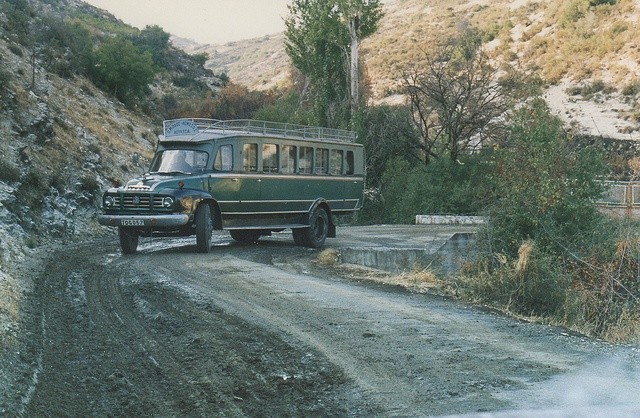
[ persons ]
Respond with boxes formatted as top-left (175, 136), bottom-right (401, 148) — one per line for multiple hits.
top-left (169, 150), bottom-right (193, 174)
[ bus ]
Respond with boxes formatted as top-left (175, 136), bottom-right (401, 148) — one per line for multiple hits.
top-left (99, 117), bottom-right (366, 255)
